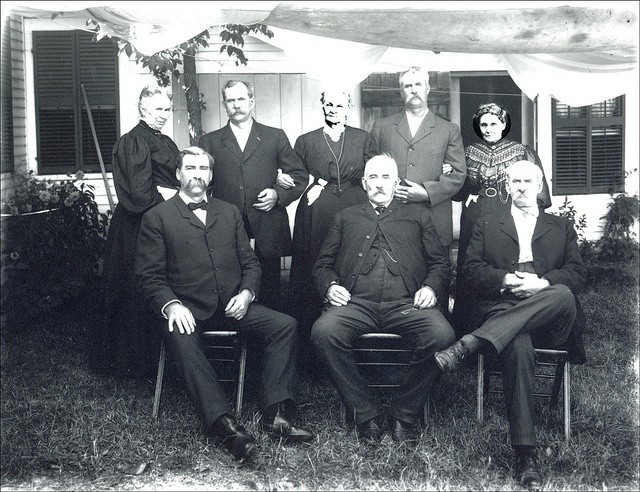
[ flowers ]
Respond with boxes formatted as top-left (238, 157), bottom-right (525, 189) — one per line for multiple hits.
top-left (2, 170), bottom-right (107, 269)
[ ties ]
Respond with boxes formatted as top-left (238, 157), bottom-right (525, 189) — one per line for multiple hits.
top-left (374, 205), bottom-right (386, 215)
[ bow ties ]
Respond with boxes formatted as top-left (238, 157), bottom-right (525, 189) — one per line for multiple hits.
top-left (187, 200), bottom-right (207, 210)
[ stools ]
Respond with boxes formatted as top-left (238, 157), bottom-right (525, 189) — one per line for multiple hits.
top-left (477, 348), bottom-right (570, 441)
top-left (340, 333), bottom-right (429, 428)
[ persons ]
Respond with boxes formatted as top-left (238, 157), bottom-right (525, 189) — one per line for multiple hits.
top-left (89, 85), bottom-right (184, 380)
top-left (200, 78), bottom-right (309, 316)
top-left (440, 101), bottom-right (550, 341)
top-left (367, 62), bottom-right (467, 321)
top-left (128, 145), bottom-right (312, 465)
top-left (433, 160), bottom-right (588, 488)
top-left (308, 152), bottom-right (455, 452)
top-left (276, 89), bottom-right (383, 381)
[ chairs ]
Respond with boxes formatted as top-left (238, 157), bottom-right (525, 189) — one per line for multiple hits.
top-left (151, 239), bottom-right (256, 420)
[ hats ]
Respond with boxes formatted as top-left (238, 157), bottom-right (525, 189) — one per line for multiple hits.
top-left (473, 104), bottom-right (512, 140)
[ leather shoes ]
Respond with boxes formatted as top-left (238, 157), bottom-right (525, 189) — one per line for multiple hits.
top-left (213, 415), bottom-right (257, 460)
top-left (388, 415), bottom-right (416, 447)
top-left (258, 406), bottom-right (312, 442)
top-left (515, 454), bottom-right (542, 487)
top-left (359, 418), bottom-right (383, 445)
top-left (435, 342), bottom-right (468, 376)
